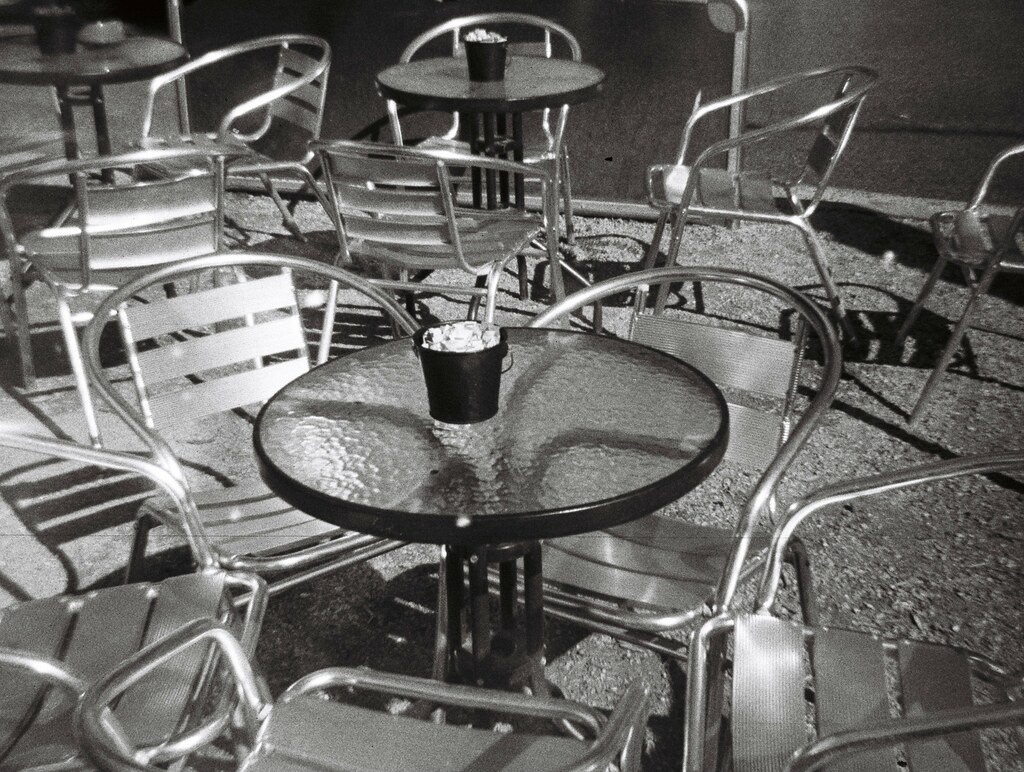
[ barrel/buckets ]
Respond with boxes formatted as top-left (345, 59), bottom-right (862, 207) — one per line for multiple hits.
top-left (33, 11), bottom-right (78, 54)
top-left (462, 36), bottom-right (511, 82)
top-left (413, 320), bottom-right (514, 425)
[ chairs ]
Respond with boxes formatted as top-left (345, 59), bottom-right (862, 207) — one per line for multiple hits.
top-left (639, 63), bottom-right (886, 352)
top-left (1, 134), bottom-right (99, 391)
top-left (1, 434), bottom-right (269, 772)
top-left (78, 615), bottom-right (652, 772)
top-left (80, 252), bottom-right (430, 712)
top-left (2, 142), bottom-right (255, 400)
top-left (682, 452), bottom-right (1023, 772)
top-left (888, 140), bottom-right (1024, 428)
top-left (136, 34), bottom-right (361, 244)
top-left (437, 263), bottom-right (845, 709)
top-left (305, 138), bottom-right (575, 339)
top-left (384, 12), bottom-right (584, 243)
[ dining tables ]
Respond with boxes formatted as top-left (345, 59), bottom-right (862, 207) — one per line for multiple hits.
top-left (374, 56), bottom-right (606, 299)
top-left (1, 33), bottom-right (189, 190)
top-left (253, 327), bottom-right (730, 743)
